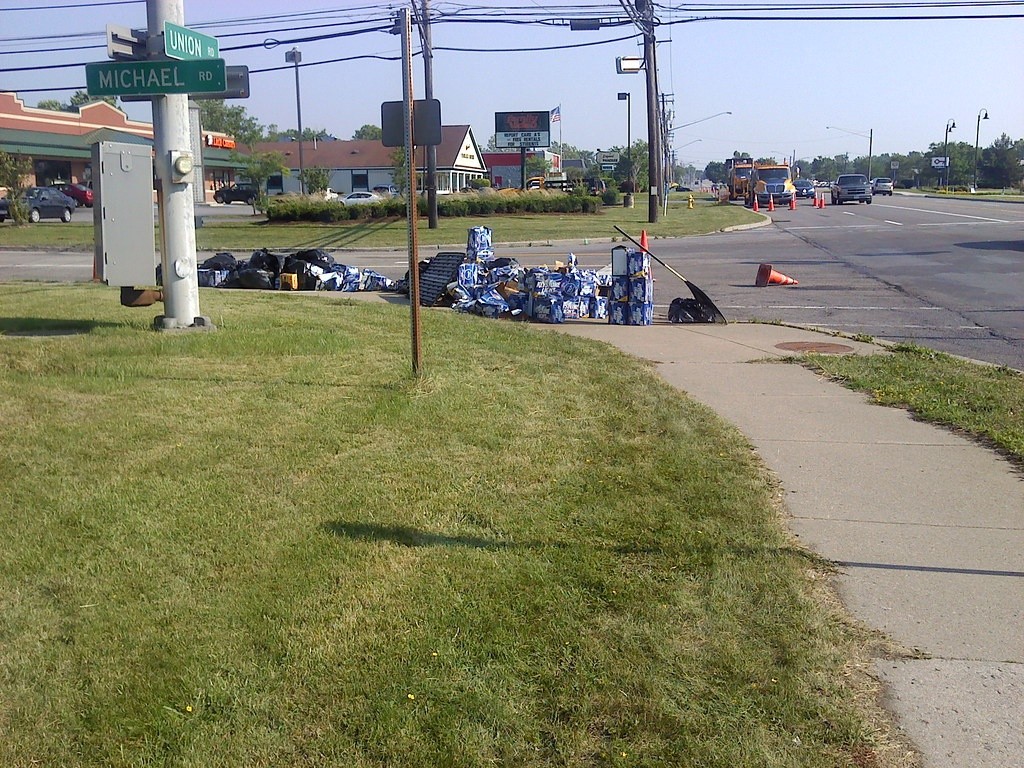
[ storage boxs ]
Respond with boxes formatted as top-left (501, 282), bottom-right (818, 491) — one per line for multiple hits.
top-left (198, 263), bottom-right (394, 292)
top-left (445, 225), bottom-right (654, 327)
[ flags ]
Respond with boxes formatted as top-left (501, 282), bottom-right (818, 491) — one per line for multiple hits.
top-left (550, 106), bottom-right (560, 122)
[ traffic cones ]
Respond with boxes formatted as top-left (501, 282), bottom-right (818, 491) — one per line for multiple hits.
top-left (639, 231), bottom-right (656, 282)
top-left (753, 193), bottom-right (826, 212)
top-left (755, 265), bottom-right (799, 285)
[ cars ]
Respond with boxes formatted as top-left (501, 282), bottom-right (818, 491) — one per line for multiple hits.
top-left (337, 192), bottom-right (382, 204)
top-left (313, 189), bottom-right (340, 200)
top-left (48, 184), bottom-right (94, 208)
top-left (81, 181), bottom-right (93, 189)
top-left (792, 180), bottom-right (815, 199)
top-left (0, 188), bottom-right (76, 223)
top-left (813, 182), bottom-right (835, 187)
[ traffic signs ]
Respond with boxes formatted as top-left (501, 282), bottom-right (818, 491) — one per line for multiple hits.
top-left (85, 58), bottom-right (227, 96)
top-left (163, 21), bottom-right (219, 60)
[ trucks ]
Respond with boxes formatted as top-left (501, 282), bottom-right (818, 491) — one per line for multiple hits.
top-left (745, 164), bottom-right (797, 205)
top-left (725, 158), bottom-right (755, 200)
top-left (519, 177), bottom-right (606, 195)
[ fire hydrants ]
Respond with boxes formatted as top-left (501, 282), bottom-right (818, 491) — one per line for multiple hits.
top-left (687, 194), bottom-right (695, 209)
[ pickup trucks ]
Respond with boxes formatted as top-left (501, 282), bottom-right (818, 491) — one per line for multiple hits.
top-left (830, 173), bottom-right (872, 205)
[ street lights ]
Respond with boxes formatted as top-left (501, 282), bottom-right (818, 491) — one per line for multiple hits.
top-left (827, 127), bottom-right (871, 184)
top-left (285, 50), bottom-right (306, 194)
top-left (974, 108), bottom-right (990, 186)
top-left (618, 92), bottom-right (632, 195)
top-left (943, 118), bottom-right (957, 188)
top-left (664, 111), bottom-right (732, 186)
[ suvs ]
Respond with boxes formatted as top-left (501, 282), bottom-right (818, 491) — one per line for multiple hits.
top-left (213, 183), bottom-right (261, 205)
top-left (373, 184), bottom-right (399, 198)
top-left (871, 178), bottom-right (893, 196)
